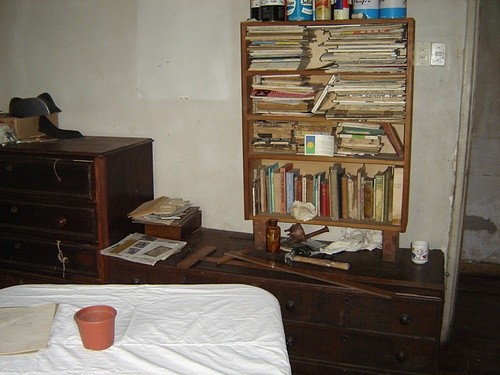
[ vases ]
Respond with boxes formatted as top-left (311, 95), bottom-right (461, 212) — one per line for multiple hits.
top-left (74, 305), bottom-right (118, 351)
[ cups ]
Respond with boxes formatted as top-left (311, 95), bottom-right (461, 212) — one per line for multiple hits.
top-left (75, 305), bottom-right (117, 351)
top-left (411, 241), bottom-right (429, 265)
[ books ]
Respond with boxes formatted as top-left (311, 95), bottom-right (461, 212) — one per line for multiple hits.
top-left (246, 25), bottom-right (408, 225)
top-left (127, 196), bottom-right (192, 226)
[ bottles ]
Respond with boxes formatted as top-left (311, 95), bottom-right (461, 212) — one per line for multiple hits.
top-left (266, 219), bottom-right (281, 254)
top-left (249, 0), bottom-right (408, 21)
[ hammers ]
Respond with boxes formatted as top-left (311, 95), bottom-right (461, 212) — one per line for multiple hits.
top-left (284, 252), bottom-right (351, 270)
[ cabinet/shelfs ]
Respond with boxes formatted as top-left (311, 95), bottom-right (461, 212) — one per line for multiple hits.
top-left (0, 133), bottom-right (155, 285)
top-left (108, 222), bottom-right (446, 374)
top-left (238, 17), bottom-right (417, 263)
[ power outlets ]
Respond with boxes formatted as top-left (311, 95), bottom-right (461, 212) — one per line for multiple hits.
top-left (430, 42), bottom-right (448, 67)
top-left (414, 41), bottom-right (431, 68)
